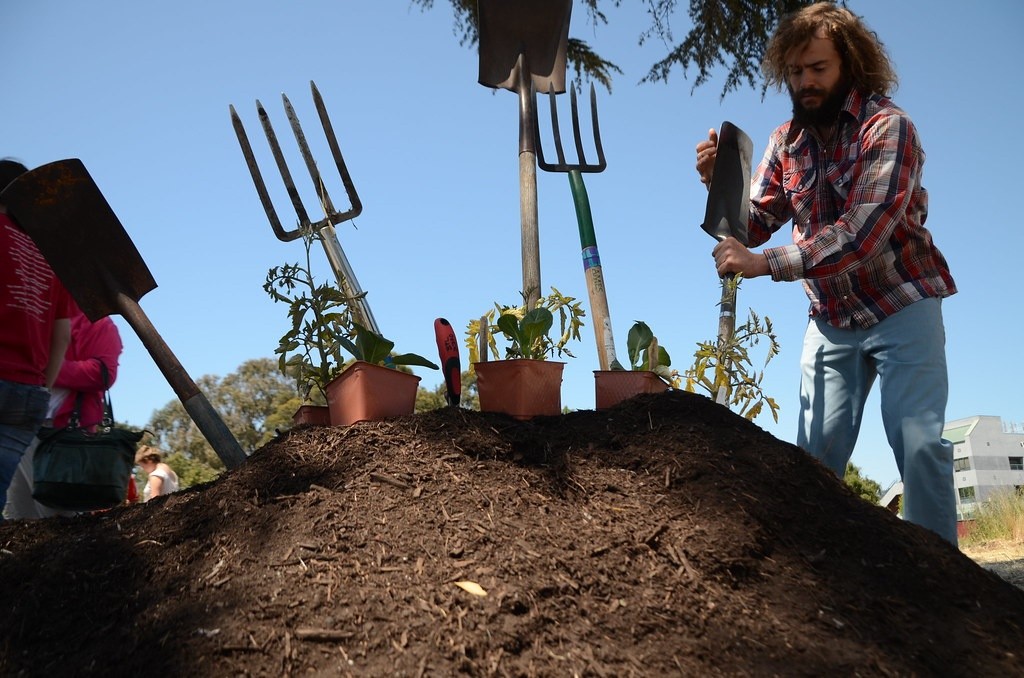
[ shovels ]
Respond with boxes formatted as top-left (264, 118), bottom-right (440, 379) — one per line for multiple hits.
top-left (470, 0), bottom-right (573, 360)
top-left (701, 121), bottom-right (755, 405)
top-left (0, 157), bottom-right (250, 472)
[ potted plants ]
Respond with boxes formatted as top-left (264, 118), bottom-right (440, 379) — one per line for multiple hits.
top-left (321, 321), bottom-right (439, 425)
top-left (592, 321), bottom-right (672, 410)
top-left (470, 307), bottom-right (568, 422)
top-left (262, 220), bottom-right (368, 425)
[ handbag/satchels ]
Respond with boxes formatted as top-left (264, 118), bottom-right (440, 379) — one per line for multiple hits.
top-left (31, 425), bottom-right (147, 511)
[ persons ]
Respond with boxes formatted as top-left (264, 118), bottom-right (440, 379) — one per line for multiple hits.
top-left (0, 157), bottom-right (180, 517)
top-left (695, 2), bottom-right (959, 547)
